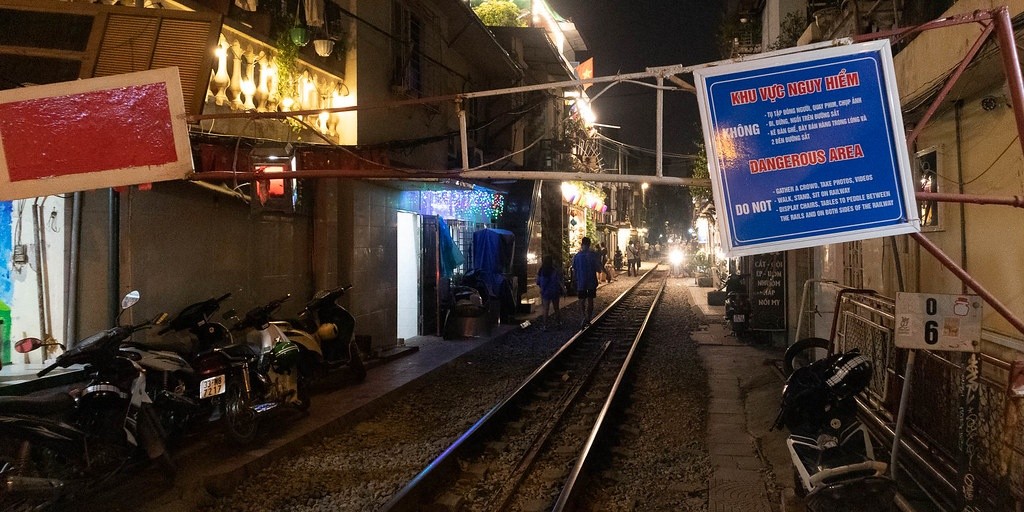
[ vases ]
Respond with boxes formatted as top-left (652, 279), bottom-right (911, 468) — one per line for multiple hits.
top-left (312, 39), bottom-right (336, 57)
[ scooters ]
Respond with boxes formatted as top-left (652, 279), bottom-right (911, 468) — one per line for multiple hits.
top-left (718, 270), bottom-right (751, 340)
top-left (114, 282), bottom-right (235, 443)
top-left (193, 294), bottom-right (309, 447)
top-left (269, 283), bottom-right (367, 393)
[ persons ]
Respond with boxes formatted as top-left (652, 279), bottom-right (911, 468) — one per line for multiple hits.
top-left (647, 242), bottom-right (665, 259)
top-left (537, 255), bottom-right (566, 324)
top-left (626, 240), bottom-right (643, 277)
top-left (573, 236), bottom-right (611, 322)
top-left (666, 243), bottom-right (686, 277)
top-left (596, 242), bottom-right (608, 284)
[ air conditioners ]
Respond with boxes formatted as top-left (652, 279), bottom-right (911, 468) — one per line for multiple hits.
top-left (448, 136), bottom-right (458, 163)
top-left (468, 147), bottom-right (484, 168)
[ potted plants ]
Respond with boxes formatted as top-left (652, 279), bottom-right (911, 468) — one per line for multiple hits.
top-left (808, 0), bottom-right (841, 34)
top-left (697, 260), bottom-right (713, 288)
top-left (681, 254), bottom-right (707, 285)
top-left (275, 26), bottom-right (313, 99)
top-left (707, 259), bottom-right (728, 306)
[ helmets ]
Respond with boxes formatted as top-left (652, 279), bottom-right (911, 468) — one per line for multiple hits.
top-left (274, 342), bottom-right (302, 368)
top-left (318, 322), bottom-right (338, 342)
top-left (826, 348), bottom-right (872, 398)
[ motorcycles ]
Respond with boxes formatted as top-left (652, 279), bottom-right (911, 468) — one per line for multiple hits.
top-left (0, 290), bottom-right (168, 511)
